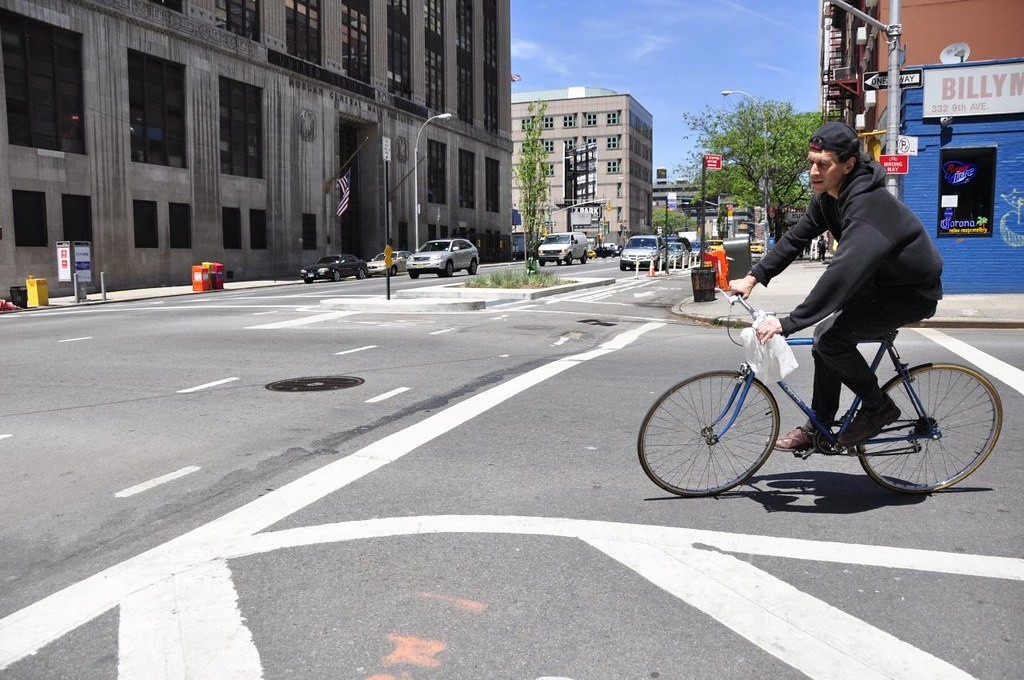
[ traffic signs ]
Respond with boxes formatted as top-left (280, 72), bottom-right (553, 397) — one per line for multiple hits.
top-left (862, 67), bottom-right (924, 91)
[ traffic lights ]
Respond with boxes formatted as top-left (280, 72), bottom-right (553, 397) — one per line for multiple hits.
top-left (607, 202), bottom-right (612, 211)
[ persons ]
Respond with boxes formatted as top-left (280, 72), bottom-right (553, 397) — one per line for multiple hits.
top-left (728, 120), bottom-right (944, 452)
top-left (818, 235), bottom-right (828, 262)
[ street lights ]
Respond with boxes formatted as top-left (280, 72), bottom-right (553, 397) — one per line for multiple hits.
top-left (673, 202), bottom-right (689, 235)
top-left (721, 90), bottom-right (768, 257)
top-left (415, 113), bottom-right (452, 252)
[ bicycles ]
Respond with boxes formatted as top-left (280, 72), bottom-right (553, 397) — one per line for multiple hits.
top-left (637, 288), bottom-right (1004, 497)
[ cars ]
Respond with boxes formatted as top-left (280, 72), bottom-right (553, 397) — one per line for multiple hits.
top-left (665, 234), bottom-right (723, 268)
top-left (750, 237), bottom-right (774, 253)
top-left (587, 246), bottom-right (620, 259)
top-left (367, 250), bottom-right (413, 278)
top-left (301, 254), bottom-right (367, 283)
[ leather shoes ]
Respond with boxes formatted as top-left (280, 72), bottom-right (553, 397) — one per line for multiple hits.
top-left (837, 392), bottom-right (901, 446)
top-left (767, 426), bottom-right (829, 452)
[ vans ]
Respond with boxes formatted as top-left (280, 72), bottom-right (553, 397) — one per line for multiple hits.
top-left (538, 232), bottom-right (588, 266)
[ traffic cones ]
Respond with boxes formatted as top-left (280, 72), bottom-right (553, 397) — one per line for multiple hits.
top-left (646, 257), bottom-right (658, 277)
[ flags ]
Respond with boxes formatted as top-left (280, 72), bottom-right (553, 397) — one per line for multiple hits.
top-left (335, 170), bottom-right (350, 216)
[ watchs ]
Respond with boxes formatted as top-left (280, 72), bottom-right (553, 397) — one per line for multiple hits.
top-left (747, 271), bottom-right (759, 282)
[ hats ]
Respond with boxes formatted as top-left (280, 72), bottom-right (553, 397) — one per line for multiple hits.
top-left (808, 121), bottom-right (871, 162)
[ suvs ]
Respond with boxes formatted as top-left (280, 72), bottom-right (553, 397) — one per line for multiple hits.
top-left (620, 234), bottom-right (667, 271)
top-left (406, 238), bottom-right (480, 279)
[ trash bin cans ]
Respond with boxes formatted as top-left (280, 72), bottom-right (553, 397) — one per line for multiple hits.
top-left (691, 267), bottom-right (716, 303)
top-left (10, 285), bottom-right (28, 309)
